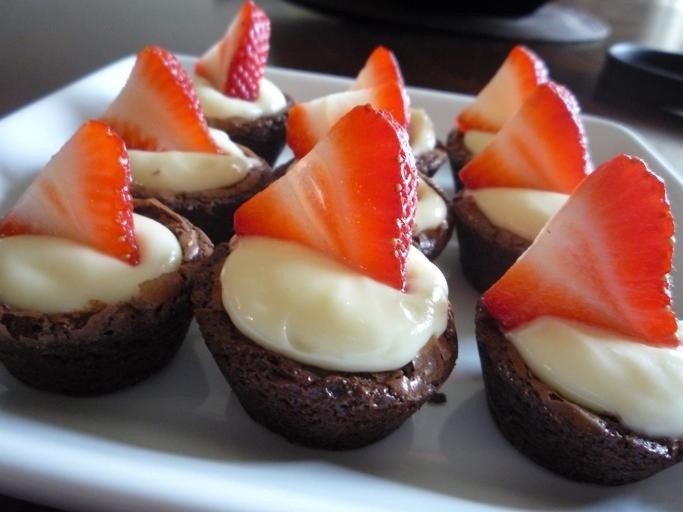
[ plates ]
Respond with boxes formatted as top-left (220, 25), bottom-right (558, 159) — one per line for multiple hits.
top-left (1, 52), bottom-right (682, 511)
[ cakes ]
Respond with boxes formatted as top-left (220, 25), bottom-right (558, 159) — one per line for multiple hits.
top-left (0, 120), bottom-right (215, 398)
top-left (98, 44), bottom-right (274, 244)
top-left (191, 103), bottom-right (458, 452)
top-left (190, 1), bottom-right (294, 171)
top-left (267, 82), bottom-right (457, 263)
top-left (475, 154), bottom-right (682, 486)
top-left (346, 43), bottom-right (449, 177)
top-left (447, 44), bottom-right (551, 168)
top-left (456, 79), bottom-right (595, 293)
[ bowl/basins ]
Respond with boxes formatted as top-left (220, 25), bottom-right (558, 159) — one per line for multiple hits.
top-left (604, 41), bottom-right (681, 117)
